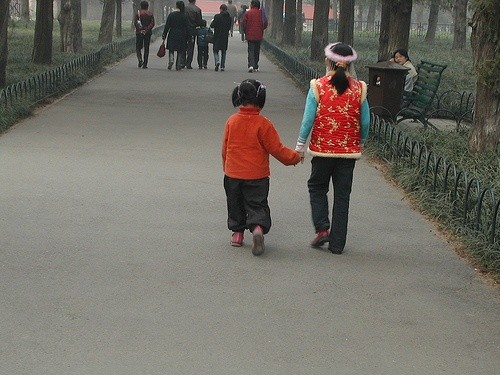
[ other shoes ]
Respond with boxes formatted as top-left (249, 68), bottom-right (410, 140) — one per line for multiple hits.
top-left (215, 63), bottom-right (220, 71)
top-left (139, 62), bottom-right (142, 67)
top-left (311, 231), bottom-right (330, 246)
top-left (143, 67), bottom-right (147, 68)
top-left (187, 67), bottom-right (192, 69)
top-left (199, 66), bottom-right (202, 69)
top-left (248, 67), bottom-right (253, 72)
top-left (254, 69), bottom-right (259, 72)
top-left (252, 226), bottom-right (265, 256)
top-left (230, 232), bottom-right (243, 246)
top-left (221, 68), bottom-right (224, 70)
top-left (204, 65), bottom-right (207, 69)
top-left (167, 62), bottom-right (173, 69)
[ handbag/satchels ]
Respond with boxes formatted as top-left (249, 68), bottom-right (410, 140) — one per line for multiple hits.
top-left (157, 41), bottom-right (165, 58)
top-left (205, 27), bottom-right (214, 43)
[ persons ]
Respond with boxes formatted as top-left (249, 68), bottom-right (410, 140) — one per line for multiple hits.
top-left (294, 42), bottom-right (370, 253)
top-left (196, 19), bottom-right (213, 69)
top-left (163, 2), bottom-right (187, 71)
top-left (390, 49), bottom-right (417, 96)
top-left (210, 4), bottom-right (232, 71)
top-left (243, 0), bottom-right (268, 72)
top-left (238, 5), bottom-right (249, 41)
top-left (182, 0), bottom-right (202, 69)
top-left (222, 79), bottom-right (304, 255)
top-left (134, 1), bottom-right (155, 68)
top-left (226, 0), bottom-right (237, 37)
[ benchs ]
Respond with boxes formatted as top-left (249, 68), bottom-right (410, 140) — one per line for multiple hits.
top-left (397, 60), bottom-right (448, 131)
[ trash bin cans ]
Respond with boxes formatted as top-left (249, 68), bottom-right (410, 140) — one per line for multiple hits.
top-left (363, 60), bottom-right (411, 125)
